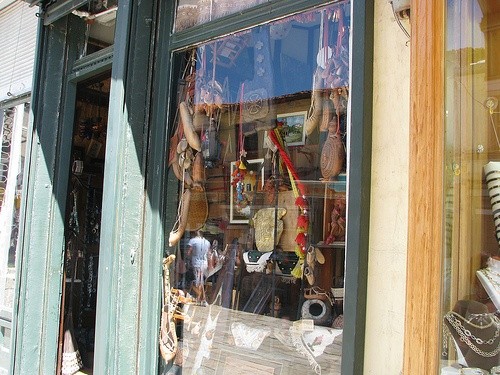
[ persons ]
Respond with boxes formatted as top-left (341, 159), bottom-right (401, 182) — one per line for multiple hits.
top-left (206, 240), bottom-right (219, 289)
top-left (186, 230), bottom-right (208, 306)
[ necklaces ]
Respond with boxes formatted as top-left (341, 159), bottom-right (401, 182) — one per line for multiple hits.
top-left (441, 311), bottom-right (500, 358)
top-left (481, 266), bottom-right (500, 292)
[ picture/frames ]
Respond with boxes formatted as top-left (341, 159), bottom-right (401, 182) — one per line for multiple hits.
top-left (263, 111), bottom-right (308, 148)
top-left (229, 157), bottom-right (265, 225)
